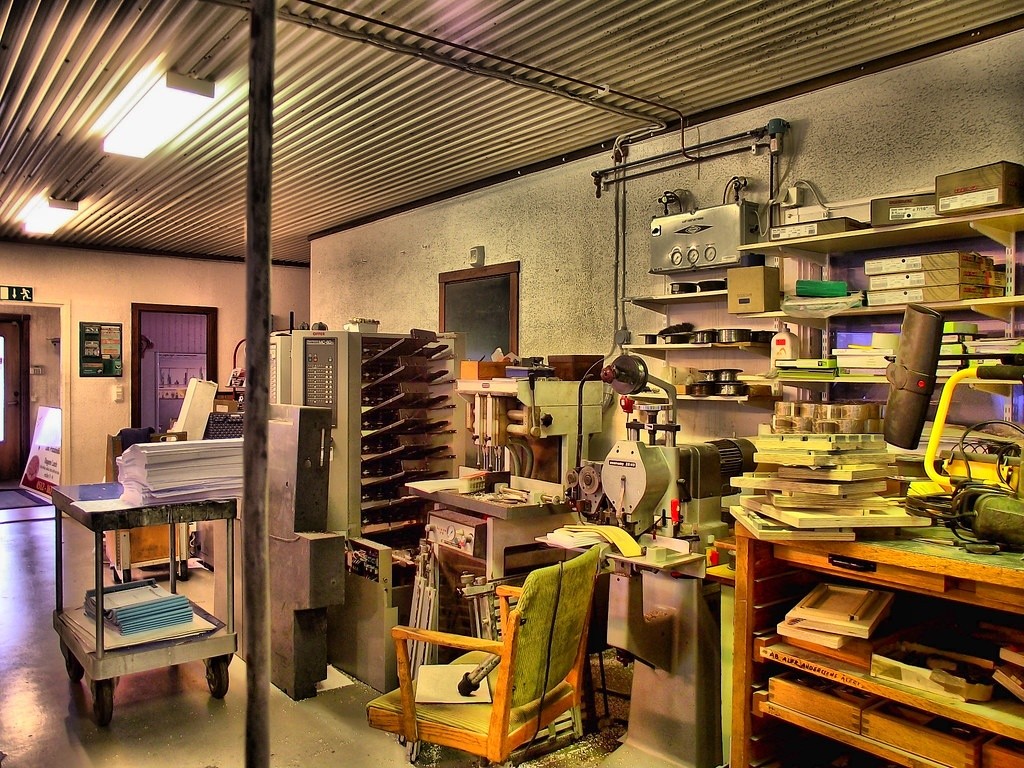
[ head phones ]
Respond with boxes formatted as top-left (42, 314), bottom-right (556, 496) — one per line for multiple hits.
top-left (905, 485), bottom-right (1024, 553)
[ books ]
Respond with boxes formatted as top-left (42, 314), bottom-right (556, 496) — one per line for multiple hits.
top-left (81, 577), bottom-right (194, 637)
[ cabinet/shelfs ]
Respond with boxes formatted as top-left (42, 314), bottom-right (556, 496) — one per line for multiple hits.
top-left (629, 289), bottom-right (764, 401)
top-left (52, 482), bottom-right (238, 728)
top-left (739, 209), bottom-right (1024, 384)
top-left (730, 519), bottom-right (1024, 768)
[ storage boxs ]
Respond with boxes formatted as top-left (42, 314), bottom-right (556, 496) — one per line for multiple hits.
top-left (548, 355), bottom-right (604, 381)
top-left (871, 160), bottom-right (1024, 229)
top-left (864, 250), bottom-right (1006, 307)
top-left (461, 361), bottom-right (514, 381)
top-left (726, 265), bottom-right (781, 314)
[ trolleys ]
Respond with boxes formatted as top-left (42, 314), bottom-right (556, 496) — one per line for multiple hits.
top-left (51, 482), bottom-right (239, 726)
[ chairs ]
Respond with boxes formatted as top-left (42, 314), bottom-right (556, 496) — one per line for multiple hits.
top-left (367, 545), bottom-right (600, 768)
